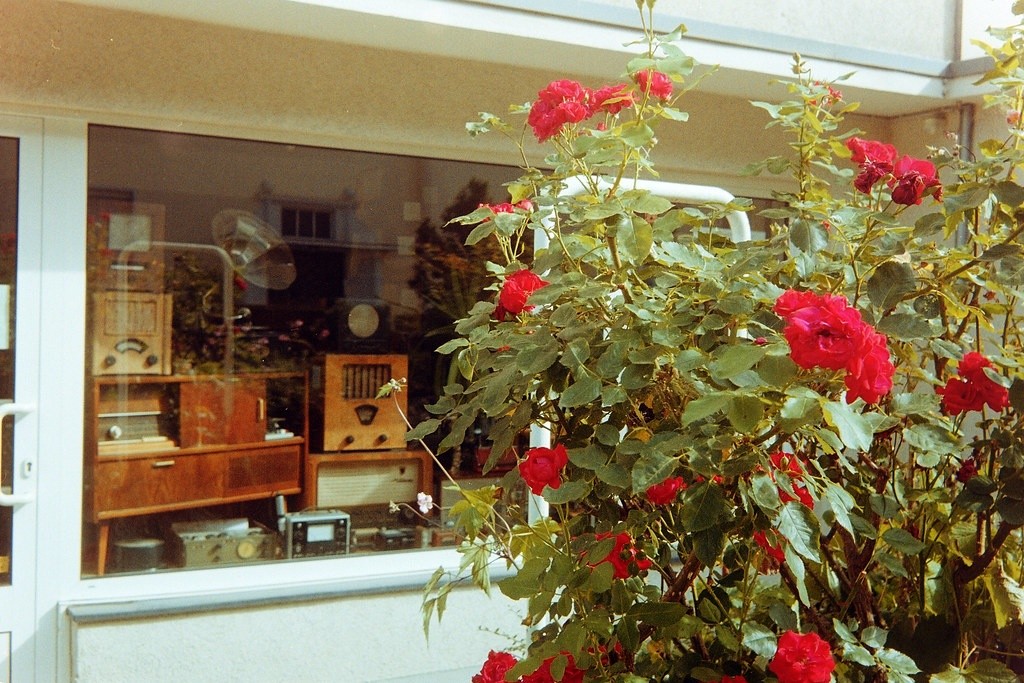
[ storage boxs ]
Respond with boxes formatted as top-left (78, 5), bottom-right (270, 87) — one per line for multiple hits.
top-left (162, 518), bottom-right (284, 567)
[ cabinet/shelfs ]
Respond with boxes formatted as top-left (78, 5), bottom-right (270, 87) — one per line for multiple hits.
top-left (83, 369), bottom-right (310, 576)
